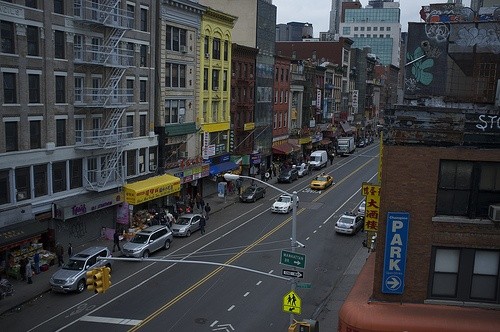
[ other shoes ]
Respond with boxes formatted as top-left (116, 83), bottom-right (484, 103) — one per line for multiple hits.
top-left (62, 261), bottom-right (64, 264)
top-left (58, 264), bottom-right (62, 267)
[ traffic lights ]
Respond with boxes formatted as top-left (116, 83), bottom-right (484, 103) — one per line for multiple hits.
top-left (95, 267), bottom-right (105, 295)
top-left (86, 267), bottom-right (99, 293)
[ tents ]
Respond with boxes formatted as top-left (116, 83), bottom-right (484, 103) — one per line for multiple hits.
top-left (124, 174), bottom-right (181, 206)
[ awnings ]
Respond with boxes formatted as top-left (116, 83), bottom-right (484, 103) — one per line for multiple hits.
top-left (272, 142), bottom-right (301, 155)
top-left (209, 160), bottom-right (239, 176)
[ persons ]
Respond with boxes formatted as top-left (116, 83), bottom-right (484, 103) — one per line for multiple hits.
top-left (18, 256), bottom-right (27, 281)
top-left (199, 215), bottom-right (206, 234)
top-left (205, 203), bottom-right (211, 220)
top-left (179, 205), bottom-right (193, 216)
top-left (34, 249), bottom-right (40, 275)
top-left (329, 153), bottom-right (334, 165)
top-left (196, 193), bottom-right (202, 210)
top-left (147, 209), bottom-right (175, 230)
top-left (55, 242), bottom-right (64, 267)
top-left (265, 162), bottom-right (277, 182)
top-left (236, 179), bottom-right (241, 195)
top-left (68, 242), bottom-right (73, 256)
top-left (25, 258), bottom-right (33, 284)
top-left (113, 230), bottom-right (121, 252)
top-left (200, 198), bottom-right (205, 212)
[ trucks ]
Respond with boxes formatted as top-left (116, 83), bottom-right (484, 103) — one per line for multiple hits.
top-left (308, 149), bottom-right (327, 169)
top-left (336, 137), bottom-right (355, 154)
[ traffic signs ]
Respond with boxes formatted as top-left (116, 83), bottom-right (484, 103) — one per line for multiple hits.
top-left (281, 270), bottom-right (304, 279)
top-left (280, 250), bottom-right (306, 269)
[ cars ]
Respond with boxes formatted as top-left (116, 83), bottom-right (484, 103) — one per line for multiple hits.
top-left (310, 172), bottom-right (334, 190)
top-left (358, 200), bottom-right (366, 218)
top-left (121, 225), bottom-right (174, 260)
top-left (171, 214), bottom-right (206, 239)
top-left (49, 245), bottom-right (112, 293)
top-left (238, 185), bottom-right (266, 203)
top-left (279, 164), bottom-right (310, 183)
top-left (356, 137), bottom-right (374, 148)
top-left (270, 193), bottom-right (300, 213)
top-left (335, 210), bottom-right (364, 235)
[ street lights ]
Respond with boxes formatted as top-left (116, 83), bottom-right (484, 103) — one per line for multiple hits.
top-left (223, 170), bottom-right (298, 332)
